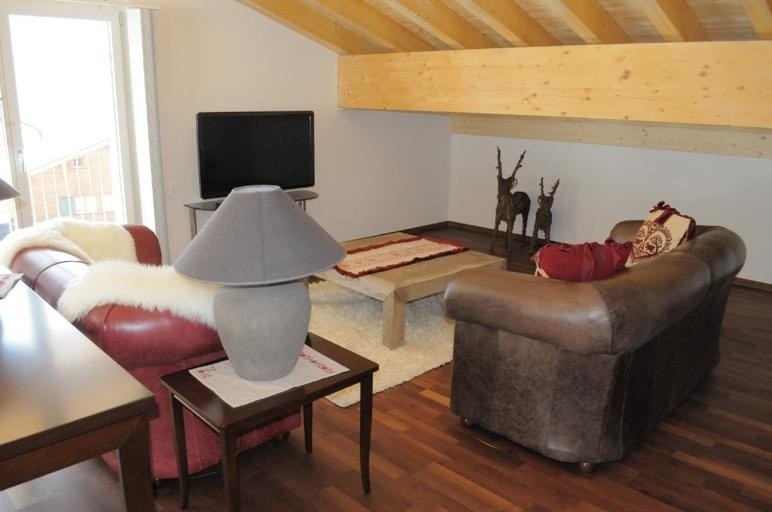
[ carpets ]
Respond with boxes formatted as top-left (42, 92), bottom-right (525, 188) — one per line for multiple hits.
top-left (307, 273), bottom-right (454, 409)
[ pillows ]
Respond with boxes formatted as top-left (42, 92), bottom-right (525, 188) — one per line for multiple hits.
top-left (532, 239), bottom-right (634, 281)
top-left (626, 201), bottom-right (696, 268)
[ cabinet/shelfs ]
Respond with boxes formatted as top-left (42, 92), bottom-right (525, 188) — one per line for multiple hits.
top-left (182, 189), bottom-right (319, 241)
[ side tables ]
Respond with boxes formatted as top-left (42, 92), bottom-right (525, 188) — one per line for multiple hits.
top-left (159, 331), bottom-right (380, 512)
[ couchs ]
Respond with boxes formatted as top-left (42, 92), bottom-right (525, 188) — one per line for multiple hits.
top-left (1, 217), bottom-right (301, 495)
top-left (440, 201), bottom-right (747, 476)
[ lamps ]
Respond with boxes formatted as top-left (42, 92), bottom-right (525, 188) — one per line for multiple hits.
top-left (0, 179), bottom-right (23, 202)
top-left (174, 186), bottom-right (347, 382)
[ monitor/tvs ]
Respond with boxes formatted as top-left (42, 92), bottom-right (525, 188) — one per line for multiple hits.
top-left (195, 111), bottom-right (315, 199)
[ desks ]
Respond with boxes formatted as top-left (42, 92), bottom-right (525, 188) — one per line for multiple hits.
top-left (0, 261), bottom-right (160, 512)
